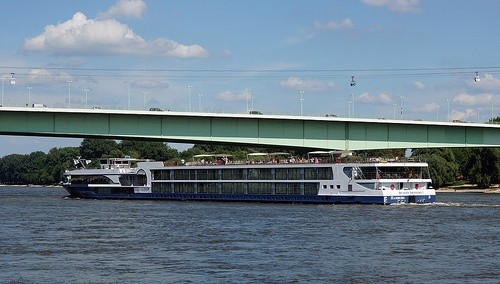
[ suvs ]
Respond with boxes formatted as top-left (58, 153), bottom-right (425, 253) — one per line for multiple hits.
top-left (250, 111), bottom-right (263, 115)
top-left (149, 108), bottom-right (162, 111)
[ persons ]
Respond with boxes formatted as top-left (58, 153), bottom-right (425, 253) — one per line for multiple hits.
top-left (171, 154), bottom-right (412, 179)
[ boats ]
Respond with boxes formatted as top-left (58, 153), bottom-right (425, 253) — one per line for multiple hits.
top-left (54, 150), bottom-right (436, 206)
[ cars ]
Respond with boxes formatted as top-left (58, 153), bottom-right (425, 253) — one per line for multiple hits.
top-left (92, 107), bottom-right (101, 109)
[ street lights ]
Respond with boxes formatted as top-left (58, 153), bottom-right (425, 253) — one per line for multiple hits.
top-left (244, 87), bottom-right (255, 113)
top-left (27, 87), bottom-right (32, 107)
top-left (198, 93), bottom-right (203, 112)
top-left (400, 96), bottom-right (404, 119)
top-left (84, 88), bottom-right (90, 109)
top-left (188, 85), bottom-right (193, 112)
top-left (392, 104), bottom-right (399, 120)
top-left (347, 102), bottom-right (352, 117)
top-left (142, 91), bottom-right (148, 110)
top-left (300, 90), bottom-right (304, 116)
top-left (446, 98), bottom-right (452, 122)
top-left (66, 81), bottom-right (72, 108)
top-left (489, 101), bottom-right (495, 124)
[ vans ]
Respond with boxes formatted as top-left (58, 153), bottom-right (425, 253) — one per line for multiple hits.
top-left (32, 103), bottom-right (47, 108)
top-left (326, 114), bottom-right (338, 117)
